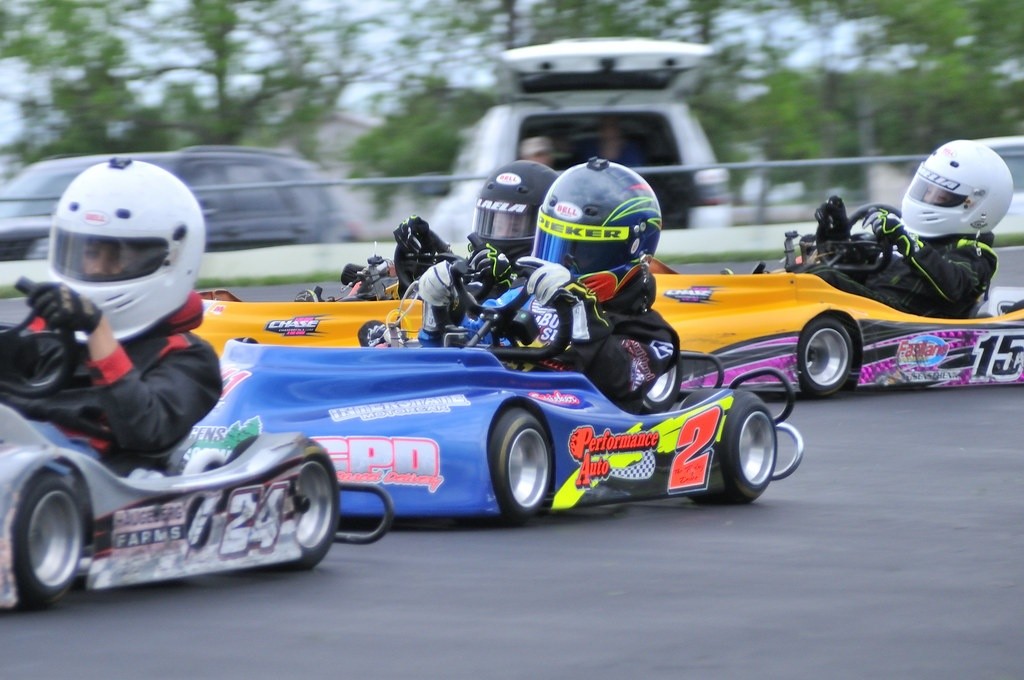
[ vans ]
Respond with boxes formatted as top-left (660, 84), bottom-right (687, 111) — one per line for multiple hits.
top-left (433, 35), bottom-right (734, 232)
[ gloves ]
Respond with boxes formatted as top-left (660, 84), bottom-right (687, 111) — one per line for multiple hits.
top-left (467, 243), bottom-right (519, 292)
top-left (815, 195), bottom-right (850, 241)
top-left (417, 260), bottom-right (461, 309)
top-left (26, 282), bottom-right (104, 335)
top-left (392, 217), bottom-right (448, 263)
top-left (516, 255), bottom-right (614, 341)
top-left (862, 207), bottom-right (926, 258)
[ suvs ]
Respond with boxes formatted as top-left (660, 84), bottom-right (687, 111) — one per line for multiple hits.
top-left (0, 142), bottom-right (362, 261)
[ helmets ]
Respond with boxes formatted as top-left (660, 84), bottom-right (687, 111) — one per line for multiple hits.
top-left (472, 159), bottom-right (559, 261)
top-left (535, 157), bottom-right (663, 301)
top-left (900, 139), bottom-right (1014, 238)
top-left (47, 157), bottom-right (207, 342)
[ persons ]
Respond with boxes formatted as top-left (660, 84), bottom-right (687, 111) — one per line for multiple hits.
top-left (721, 140), bottom-right (1012, 318)
top-left (417, 157), bottom-right (677, 411)
top-left (518, 115), bottom-right (647, 171)
top-left (293, 160), bottom-right (560, 302)
top-left (16, 161), bottom-right (222, 478)
